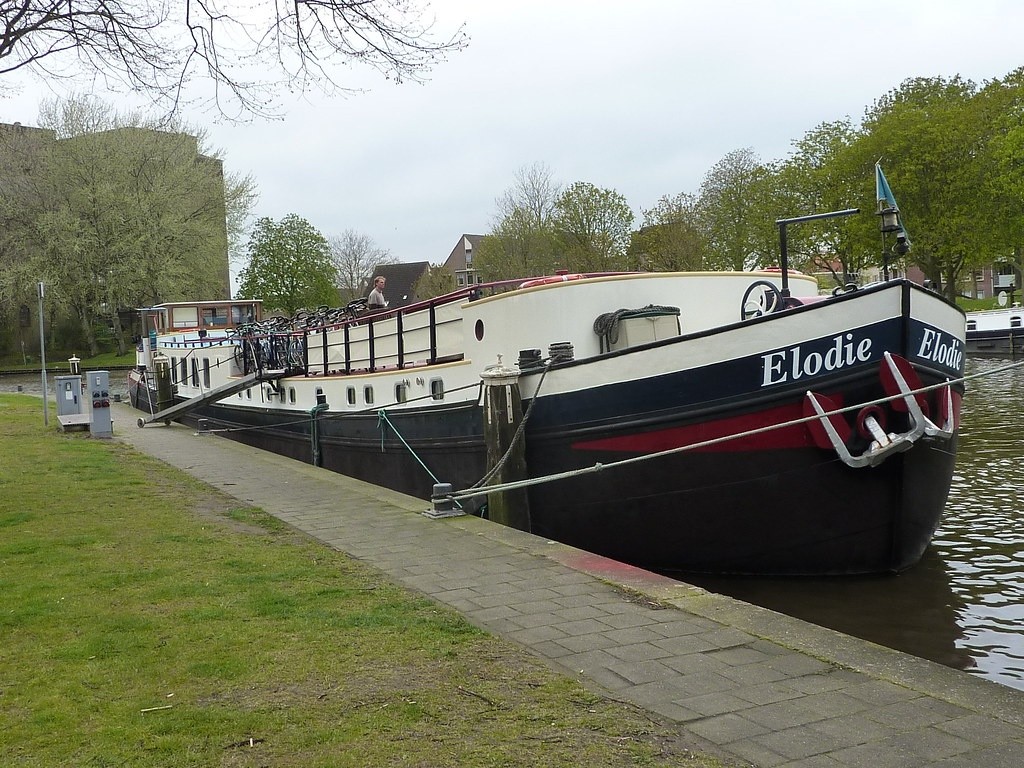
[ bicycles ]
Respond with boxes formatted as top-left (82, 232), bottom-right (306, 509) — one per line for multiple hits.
top-left (225, 297), bottom-right (371, 377)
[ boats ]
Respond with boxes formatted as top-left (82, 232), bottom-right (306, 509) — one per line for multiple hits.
top-left (127, 155), bottom-right (966, 579)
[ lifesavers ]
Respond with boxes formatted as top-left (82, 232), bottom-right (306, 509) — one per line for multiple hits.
top-left (518, 273), bottom-right (588, 290)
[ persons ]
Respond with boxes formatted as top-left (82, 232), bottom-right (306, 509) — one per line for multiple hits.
top-left (368, 275), bottom-right (386, 308)
